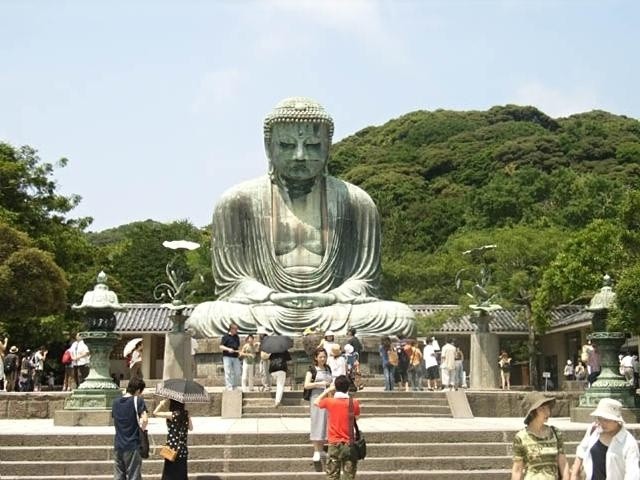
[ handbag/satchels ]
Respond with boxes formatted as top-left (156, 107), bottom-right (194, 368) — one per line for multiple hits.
top-left (4, 363), bottom-right (13, 376)
top-left (160, 445), bottom-right (179, 462)
top-left (137, 428), bottom-right (152, 459)
top-left (353, 437), bottom-right (367, 460)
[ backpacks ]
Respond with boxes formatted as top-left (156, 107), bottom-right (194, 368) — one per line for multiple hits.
top-left (502, 362), bottom-right (511, 373)
top-left (302, 387), bottom-right (311, 402)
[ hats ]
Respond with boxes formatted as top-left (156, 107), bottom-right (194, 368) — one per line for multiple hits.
top-left (322, 331), bottom-right (336, 337)
top-left (589, 397), bottom-right (626, 426)
top-left (9, 345), bottom-right (19, 353)
top-left (344, 344), bottom-right (355, 354)
top-left (329, 344), bottom-right (342, 357)
top-left (518, 390), bottom-right (558, 425)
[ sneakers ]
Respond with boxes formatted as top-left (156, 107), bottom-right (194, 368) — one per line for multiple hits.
top-left (412, 386), bottom-right (425, 391)
top-left (384, 385), bottom-right (394, 391)
top-left (427, 385), bottom-right (438, 392)
top-left (241, 386), bottom-right (255, 392)
top-left (442, 384), bottom-right (456, 393)
top-left (394, 386), bottom-right (406, 392)
top-left (264, 386), bottom-right (273, 392)
top-left (311, 449), bottom-right (329, 463)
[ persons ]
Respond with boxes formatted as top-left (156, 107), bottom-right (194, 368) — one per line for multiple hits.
top-left (512, 392), bottom-right (569, 480)
top-left (130, 344), bottom-right (143, 378)
top-left (304, 348), bottom-right (337, 462)
top-left (186, 328), bottom-right (199, 377)
top-left (184, 96), bottom-right (413, 340)
top-left (1, 333), bottom-right (89, 391)
top-left (314, 375), bottom-right (359, 480)
top-left (153, 394), bottom-right (193, 479)
top-left (220, 322), bottom-right (469, 404)
top-left (563, 344), bottom-right (640, 383)
top-left (113, 378), bottom-right (149, 479)
top-left (126, 351), bottom-right (134, 368)
top-left (497, 350), bottom-right (512, 390)
top-left (567, 397), bottom-right (640, 479)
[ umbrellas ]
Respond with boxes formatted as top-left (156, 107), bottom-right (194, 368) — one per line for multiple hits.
top-left (155, 378), bottom-right (210, 405)
top-left (123, 338), bottom-right (143, 357)
